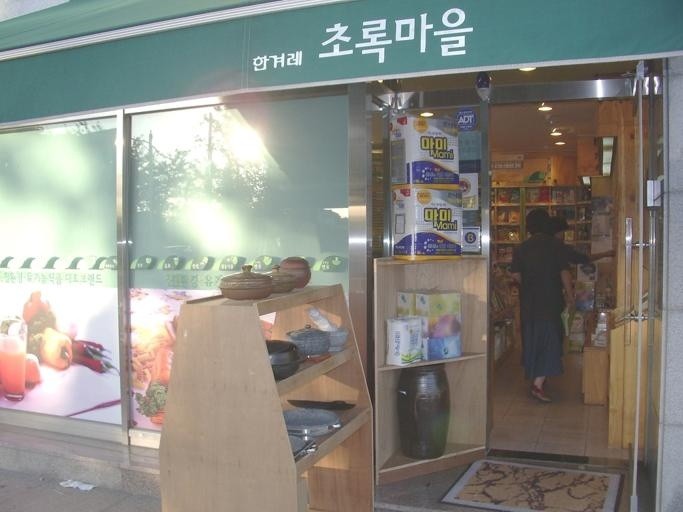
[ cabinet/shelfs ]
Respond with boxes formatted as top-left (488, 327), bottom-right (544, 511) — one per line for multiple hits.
top-left (373, 256), bottom-right (490, 484)
top-left (161, 282), bottom-right (377, 511)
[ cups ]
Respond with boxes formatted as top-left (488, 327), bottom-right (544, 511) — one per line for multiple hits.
top-left (0, 333), bottom-right (27, 400)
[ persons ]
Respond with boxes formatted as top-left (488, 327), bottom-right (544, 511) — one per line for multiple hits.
top-left (544, 216), bottom-right (593, 267)
top-left (516, 210), bottom-right (576, 403)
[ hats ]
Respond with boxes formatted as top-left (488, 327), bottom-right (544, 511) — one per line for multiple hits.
top-left (550, 215), bottom-right (573, 236)
top-left (526, 208), bottom-right (550, 235)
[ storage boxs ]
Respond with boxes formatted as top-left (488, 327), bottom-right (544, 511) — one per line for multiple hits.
top-left (387, 115), bottom-right (461, 187)
top-left (389, 183), bottom-right (464, 261)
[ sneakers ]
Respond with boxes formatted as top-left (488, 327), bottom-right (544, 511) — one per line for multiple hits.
top-left (528, 385), bottom-right (553, 404)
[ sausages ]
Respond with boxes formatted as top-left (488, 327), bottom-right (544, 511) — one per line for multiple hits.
top-left (155, 348), bottom-right (173, 385)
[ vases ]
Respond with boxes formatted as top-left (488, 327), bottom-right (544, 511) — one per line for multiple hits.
top-left (395, 364), bottom-right (452, 462)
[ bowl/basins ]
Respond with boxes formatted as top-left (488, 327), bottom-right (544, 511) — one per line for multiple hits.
top-left (282, 405), bottom-right (341, 437)
top-left (217, 263), bottom-right (296, 300)
top-left (325, 329), bottom-right (348, 352)
top-left (287, 325), bottom-right (329, 358)
top-left (268, 352), bottom-right (306, 379)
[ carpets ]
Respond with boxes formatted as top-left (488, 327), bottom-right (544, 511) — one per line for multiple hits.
top-left (439, 459), bottom-right (626, 511)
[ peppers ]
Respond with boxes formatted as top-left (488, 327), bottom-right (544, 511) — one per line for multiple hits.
top-left (23, 291), bottom-right (120, 379)
